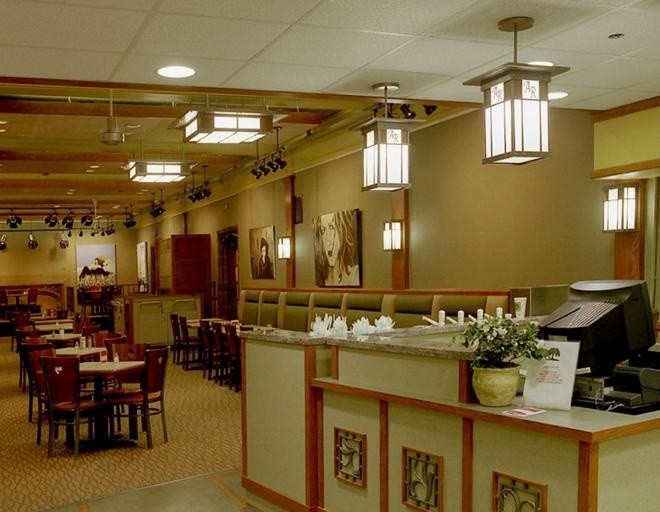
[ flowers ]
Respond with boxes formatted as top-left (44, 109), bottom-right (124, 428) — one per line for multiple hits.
top-left (448, 315), bottom-right (560, 367)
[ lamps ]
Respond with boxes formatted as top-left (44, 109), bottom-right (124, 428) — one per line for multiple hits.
top-left (349, 83), bottom-right (425, 193)
top-left (602, 183), bottom-right (637, 233)
top-left (462, 18), bottom-right (569, 166)
top-left (123, 160), bottom-right (191, 183)
top-left (382, 219), bottom-right (402, 251)
top-left (189, 164), bottom-right (211, 202)
top-left (0, 191), bottom-right (169, 250)
top-left (170, 106), bottom-right (274, 144)
top-left (249, 127), bottom-right (287, 179)
top-left (277, 236), bottom-right (290, 260)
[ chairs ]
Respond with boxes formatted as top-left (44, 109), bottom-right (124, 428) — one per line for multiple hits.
top-left (1, 284), bottom-right (253, 355)
top-left (224, 323), bottom-right (242, 392)
top-left (103, 343), bottom-right (171, 449)
top-left (20, 344), bottom-right (56, 422)
top-left (102, 341), bottom-right (148, 433)
top-left (211, 321), bottom-right (228, 387)
top-left (26, 348), bottom-right (92, 446)
top-left (39, 355), bottom-right (97, 458)
top-left (179, 316), bottom-right (198, 372)
top-left (170, 313), bottom-right (197, 366)
top-left (198, 319), bottom-right (216, 381)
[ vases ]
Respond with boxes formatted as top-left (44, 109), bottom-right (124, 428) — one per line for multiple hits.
top-left (471, 365), bottom-right (521, 406)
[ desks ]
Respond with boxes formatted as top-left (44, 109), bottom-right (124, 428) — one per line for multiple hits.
top-left (55, 361), bottom-right (146, 453)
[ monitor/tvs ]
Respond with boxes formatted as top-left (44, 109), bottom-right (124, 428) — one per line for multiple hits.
top-left (538, 278), bottom-right (656, 377)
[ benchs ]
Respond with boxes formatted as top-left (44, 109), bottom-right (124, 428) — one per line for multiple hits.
top-left (237, 289), bottom-right (509, 337)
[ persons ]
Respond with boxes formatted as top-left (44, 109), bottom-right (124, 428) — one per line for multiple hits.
top-left (255, 237), bottom-right (275, 277)
top-left (314, 210), bottom-right (359, 286)
